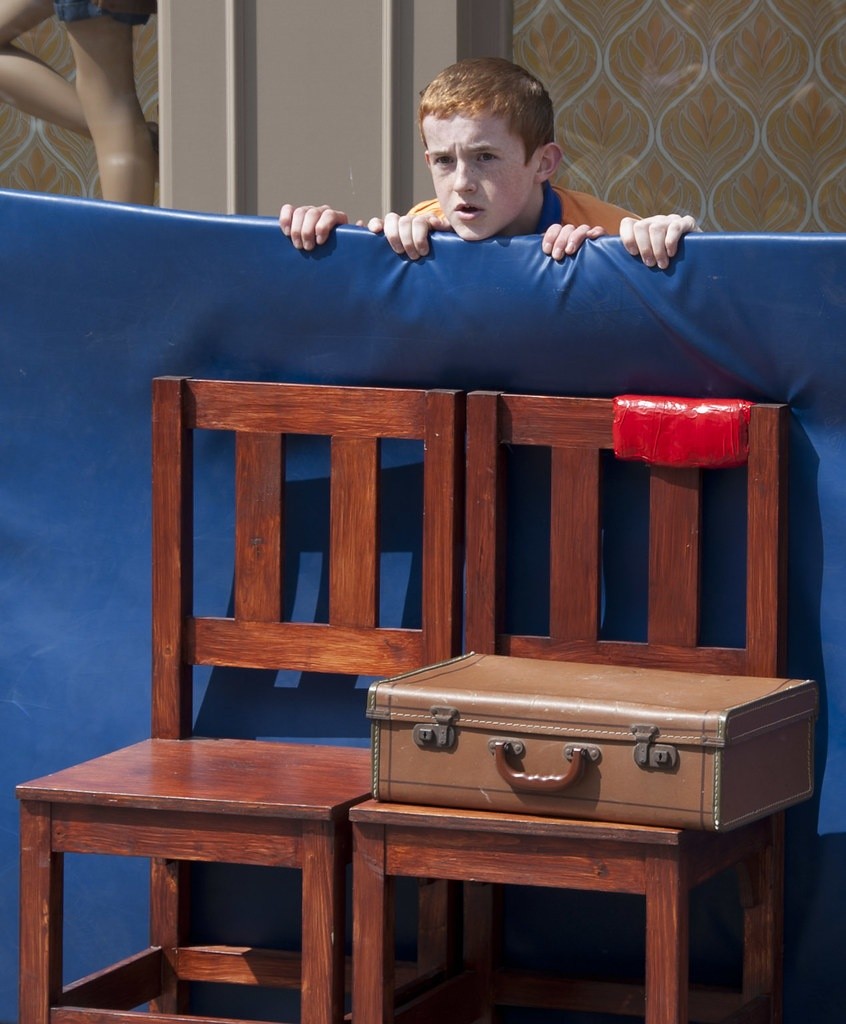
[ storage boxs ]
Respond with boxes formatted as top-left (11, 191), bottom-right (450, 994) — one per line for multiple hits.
top-left (367, 653), bottom-right (816, 833)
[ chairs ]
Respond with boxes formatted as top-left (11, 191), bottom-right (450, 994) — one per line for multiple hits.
top-left (16, 375), bottom-right (461, 1024)
top-left (348, 390), bottom-right (787, 1024)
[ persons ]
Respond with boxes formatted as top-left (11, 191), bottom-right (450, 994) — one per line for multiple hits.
top-left (277, 58), bottom-right (699, 271)
top-left (1, 0), bottom-right (158, 206)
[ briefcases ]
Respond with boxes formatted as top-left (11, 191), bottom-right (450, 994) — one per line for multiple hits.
top-left (366, 651), bottom-right (819, 832)
